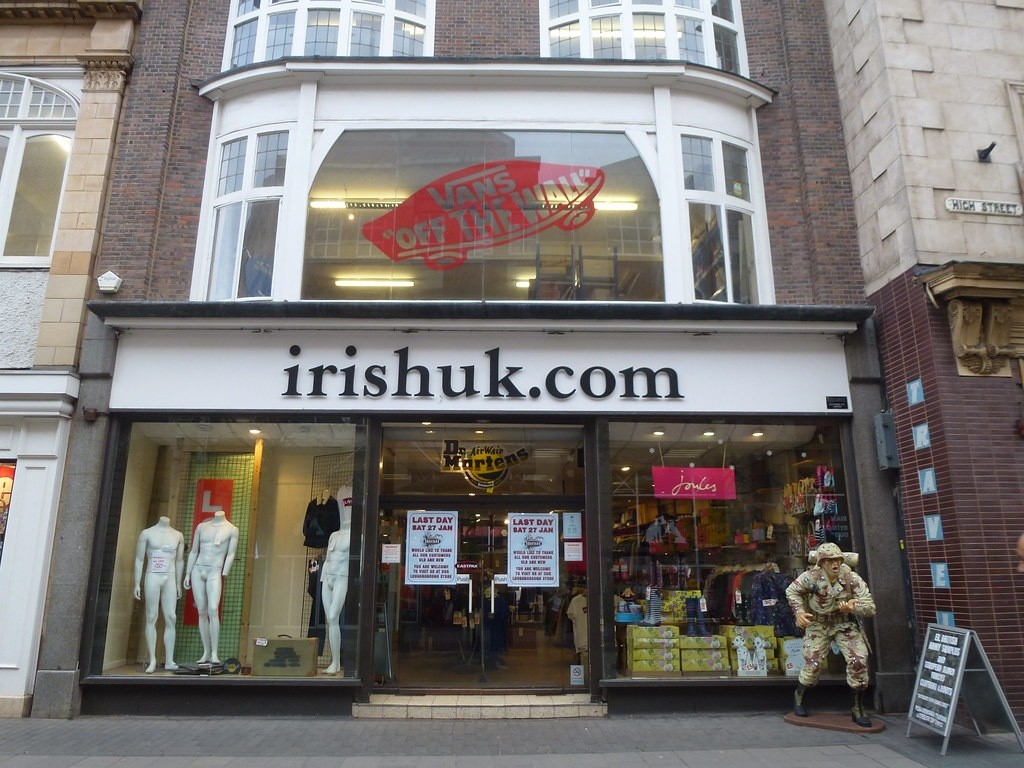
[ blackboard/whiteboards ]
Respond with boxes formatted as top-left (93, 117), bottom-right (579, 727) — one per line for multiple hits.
top-left (908, 622), bottom-right (968, 737)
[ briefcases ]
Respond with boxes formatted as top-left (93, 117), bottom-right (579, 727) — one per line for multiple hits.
top-left (251, 634), bottom-right (318, 676)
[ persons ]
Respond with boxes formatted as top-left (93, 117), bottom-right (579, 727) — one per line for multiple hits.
top-left (133, 516), bottom-right (185, 673)
top-left (321, 519), bottom-right (349, 673)
top-left (786, 543), bottom-right (876, 728)
top-left (183, 510), bottom-right (240, 664)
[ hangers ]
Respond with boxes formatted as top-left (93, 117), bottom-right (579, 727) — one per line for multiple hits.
top-left (562, 576), bottom-right (625, 604)
top-left (614, 536), bottom-right (647, 550)
top-left (708, 556), bottom-right (789, 585)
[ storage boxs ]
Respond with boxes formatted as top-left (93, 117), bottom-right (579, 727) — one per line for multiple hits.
top-left (626, 625), bottom-right (828, 678)
top-left (637, 589), bottom-right (701, 622)
top-left (511, 623), bottom-right (536, 649)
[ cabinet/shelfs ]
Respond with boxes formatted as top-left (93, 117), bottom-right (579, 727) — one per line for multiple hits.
top-left (691, 210), bottom-right (750, 304)
top-left (576, 246), bottom-right (619, 300)
top-left (529, 244), bottom-right (574, 300)
